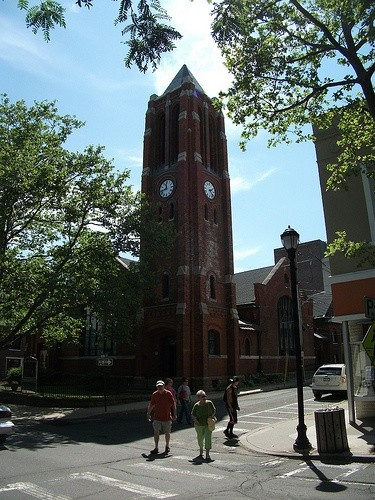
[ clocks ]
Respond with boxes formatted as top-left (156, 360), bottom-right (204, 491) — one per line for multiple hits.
top-left (203, 180), bottom-right (216, 200)
top-left (159, 180), bottom-right (174, 197)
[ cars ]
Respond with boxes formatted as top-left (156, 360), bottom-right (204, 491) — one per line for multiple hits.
top-left (0, 404), bottom-right (15, 444)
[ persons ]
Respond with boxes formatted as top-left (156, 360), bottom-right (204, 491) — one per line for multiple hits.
top-left (165, 379), bottom-right (176, 401)
top-left (177, 378), bottom-right (193, 425)
top-left (147, 380), bottom-right (177, 453)
top-left (191, 390), bottom-right (216, 460)
top-left (224, 376), bottom-right (240, 438)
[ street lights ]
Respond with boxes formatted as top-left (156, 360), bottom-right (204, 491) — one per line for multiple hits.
top-left (280, 223), bottom-right (315, 453)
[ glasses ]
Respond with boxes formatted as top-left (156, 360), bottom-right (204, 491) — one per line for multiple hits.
top-left (202, 396), bottom-right (206, 398)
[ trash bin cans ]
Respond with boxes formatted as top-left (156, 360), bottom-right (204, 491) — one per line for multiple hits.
top-left (314, 407), bottom-right (349, 454)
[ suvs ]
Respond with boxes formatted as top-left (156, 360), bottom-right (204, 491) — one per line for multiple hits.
top-left (311, 363), bottom-right (348, 399)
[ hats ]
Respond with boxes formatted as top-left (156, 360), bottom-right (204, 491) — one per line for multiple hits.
top-left (156, 380), bottom-right (165, 386)
top-left (196, 390), bottom-right (205, 396)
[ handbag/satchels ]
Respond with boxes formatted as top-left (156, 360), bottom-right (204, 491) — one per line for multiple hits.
top-left (207, 416), bottom-right (215, 432)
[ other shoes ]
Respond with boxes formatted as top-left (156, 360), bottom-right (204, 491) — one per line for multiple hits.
top-left (186, 419), bottom-right (192, 425)
top-left (150, 448), bottom-right (158, 452)
top-left (177, 418), bottom-right (183, 424)
top-left (165, 446), bottom-right (170, 452)
top-left (223, 429), bottom-right (238, 439)
